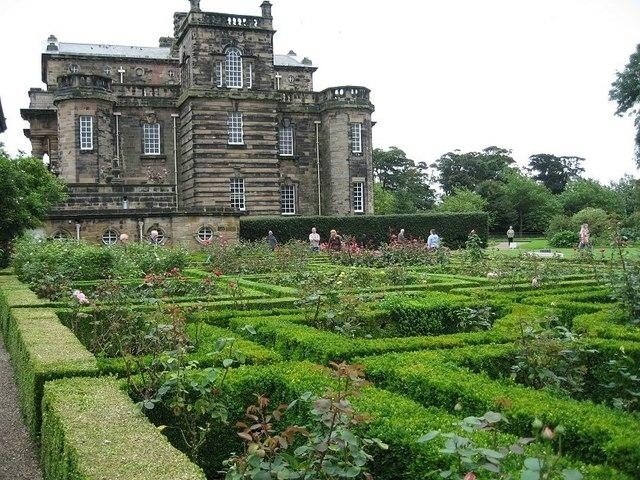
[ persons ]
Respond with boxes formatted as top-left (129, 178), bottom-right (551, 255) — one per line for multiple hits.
top-left (267, 231), bottom-right (278, 251)
top-left (329, 230), bottom-right (342, 251)
top-left (148, 231), bottom-right (158, 244)
top-left (427, 229), bottom-right (440, 246)
top-left (398, 229), bottom-right (405, 241)
top-left (309, 227), bottom-right (320, 251)
top-left (120, 234), bottom-right (129, 244)
top-left (507, 226), bottom-right (514, 245)
top-left (579, 224), bottom-right (589, 250)
